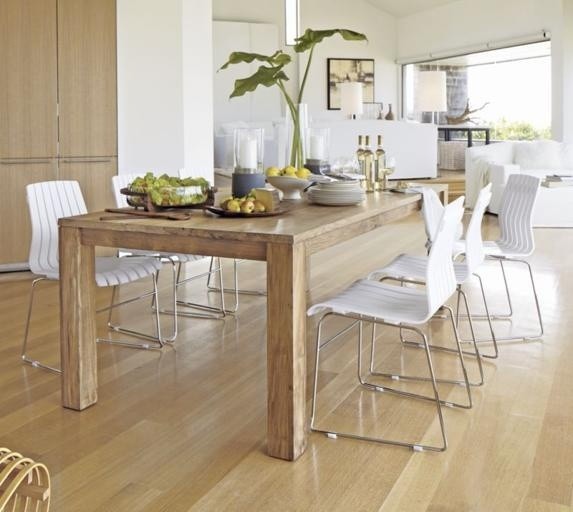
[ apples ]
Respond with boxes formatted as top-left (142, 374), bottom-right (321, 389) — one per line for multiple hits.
top-left (265, 167), bottom-right (281, 177)
top-left (281, 166), bottom-right (296, 176)
top-left (240, 201), bottom-right (255, 213)
top-left (296, 168), bottom-right (310, 179)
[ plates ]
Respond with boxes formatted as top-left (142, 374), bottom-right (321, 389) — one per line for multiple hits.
top-left (206, 205), bottom-right (285, 217)
top-left (307, 180), bottom-right (366, 206)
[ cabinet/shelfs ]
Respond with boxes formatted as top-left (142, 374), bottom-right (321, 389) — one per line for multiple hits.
top-left (0, 0), bottom-right (118, 272)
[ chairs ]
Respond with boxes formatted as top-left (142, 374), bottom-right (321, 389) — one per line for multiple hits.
top-left (175, 255), bottom-right (267, 313)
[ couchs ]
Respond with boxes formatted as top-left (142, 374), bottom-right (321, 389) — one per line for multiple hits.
top-left (464, 140), bottom-right (573, 211)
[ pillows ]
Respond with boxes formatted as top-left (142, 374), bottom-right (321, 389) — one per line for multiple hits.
top-left (514, 141), bottom-right (562, 169)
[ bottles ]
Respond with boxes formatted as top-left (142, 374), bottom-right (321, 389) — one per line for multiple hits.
top-left (358, 135), bottom-right (388, 192)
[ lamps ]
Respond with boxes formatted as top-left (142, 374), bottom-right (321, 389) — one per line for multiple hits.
top-left (417, 70), bottom-right (448, 123)
top-left (339, 82), bottom-right (363, 119)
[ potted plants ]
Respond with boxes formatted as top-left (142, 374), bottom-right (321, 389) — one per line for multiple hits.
top-left (216, 28), bottom-right (368, 171)
